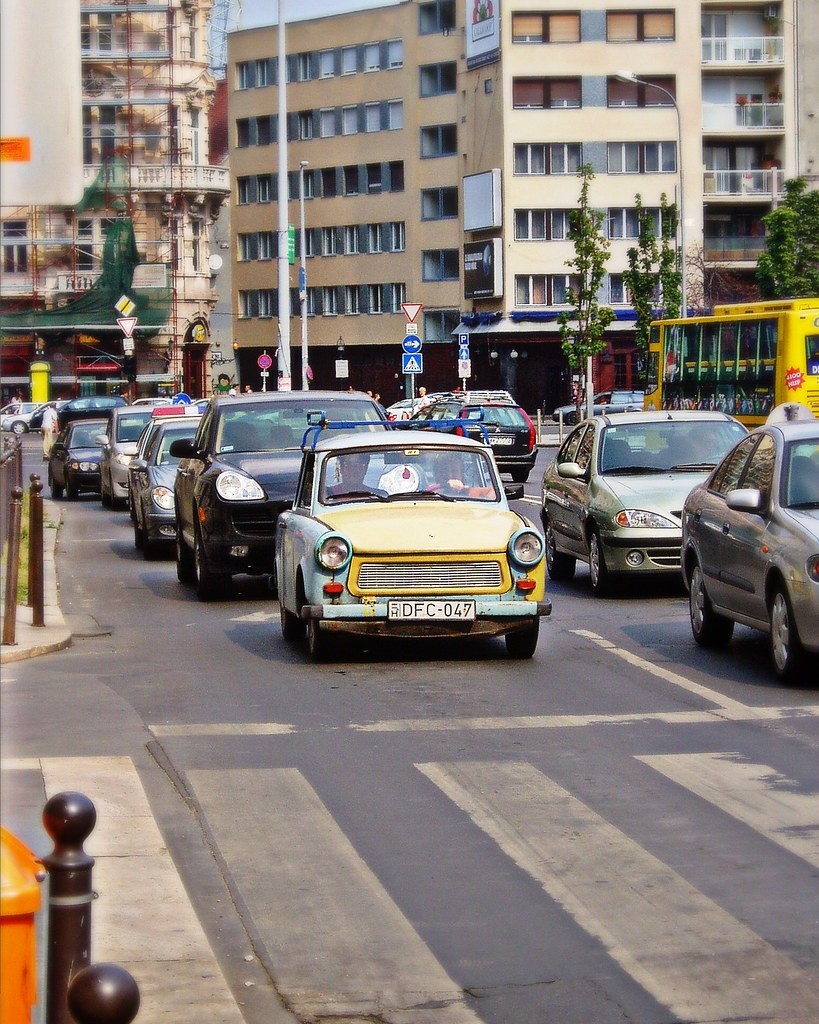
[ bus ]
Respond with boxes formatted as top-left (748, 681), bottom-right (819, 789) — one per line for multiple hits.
top-left (642, 297), bottom-right (819, 458)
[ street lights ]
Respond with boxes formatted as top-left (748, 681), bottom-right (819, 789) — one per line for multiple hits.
top-left (612, 69), bottom-right (689, 317)
top-left (297, 160), bottom-right (311, 389)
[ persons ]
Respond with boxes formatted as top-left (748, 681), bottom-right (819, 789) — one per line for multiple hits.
top-left (367, 391), bottom-right (372, 397)
top-left (41, 402), bottom-right (61, 461)
top-left (599, 396), bottom-right (607, 405)
top-left (419, 386), bottom-right (428, 396)
top-left (11, 393), bottom-right (22, 411)
top-left (245, 385), bottom-right (253, 393)
top-left (229, 383), bottom-right (239, 395)
top-left (375, 393), bottom-right (381, 401)
top-left (328, 454), bottom-right (389, 498)
top-left (431, 454), bottom-right (497, 499)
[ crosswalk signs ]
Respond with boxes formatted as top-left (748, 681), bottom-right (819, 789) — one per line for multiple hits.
top-left (402, 353), bottom-right (424, 374)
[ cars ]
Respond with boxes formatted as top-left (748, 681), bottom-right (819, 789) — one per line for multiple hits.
top-left (681, 418), bottom-right (819, 688)
top-left (0, 386), bottom-right (538, 602)
top-left (272, 408), bottom-right (553, 662)
top-left (539, 410), bottom-right (752, 595)
top-left (552, 390), bottom-right (644, 425)
